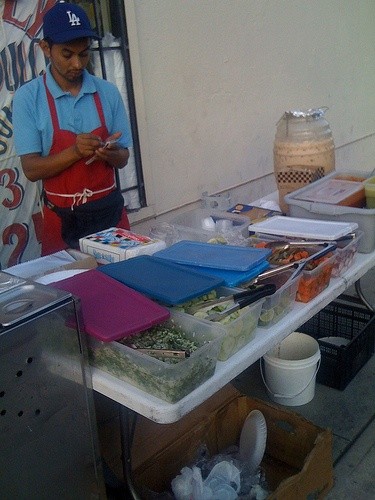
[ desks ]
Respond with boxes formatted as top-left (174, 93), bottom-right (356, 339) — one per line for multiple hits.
top-left (23, 171), bottom-right (374, 500)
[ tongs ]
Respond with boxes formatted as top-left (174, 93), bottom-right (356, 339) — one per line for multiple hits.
top-left (275, 241), bottom-right (338, 262)
top-left (238, 259), bottom-right (310, 289)
top-left (184, 283), bottom-right (276, 323)
top-left (132, 349), bottom-right (188, 359)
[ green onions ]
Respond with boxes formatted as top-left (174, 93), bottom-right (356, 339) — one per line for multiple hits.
top-left (84, 320), bottom-right (217, 404)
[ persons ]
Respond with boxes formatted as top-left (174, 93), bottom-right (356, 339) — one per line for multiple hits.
top-left (11, 0), bottom-right (134, 257)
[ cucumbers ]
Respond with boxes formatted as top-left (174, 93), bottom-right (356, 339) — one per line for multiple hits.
top-left (165, 289), bottom-right (261, 360)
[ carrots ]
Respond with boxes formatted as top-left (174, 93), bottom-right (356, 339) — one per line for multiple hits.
top-left (256, 242), bottom-right (334, 303)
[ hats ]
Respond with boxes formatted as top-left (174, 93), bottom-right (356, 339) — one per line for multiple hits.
top-left (43, 2), bottom-right (101, 43)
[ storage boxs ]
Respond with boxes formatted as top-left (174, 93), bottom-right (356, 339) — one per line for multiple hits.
top-left (217, 266), bottom-right (304, 328)
top-left (138, 286), bottom-right (264, 349)
top-left (277, 165), bottom-right (325, 215)
top-left (62, 308), bottom-right (228, 403)
top-left (237, 238), bottom-right (338, 303)
top-left (255, 222), bottom-right (364, 278)
top-left (285, 167), bottom-right (375, 254)
top-left (103, 386), bottom-right (334, 500)
top-left (294, 303), bottom-right (375, 390)
top-left (158, 207), bottom-right (250, 247)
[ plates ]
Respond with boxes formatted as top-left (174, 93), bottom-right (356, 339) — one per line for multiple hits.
top-left (239, 409), bottom-right (267, 470)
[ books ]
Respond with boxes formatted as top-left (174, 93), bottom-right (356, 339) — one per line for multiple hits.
top-left (84, 137), bottom-right (121, 167)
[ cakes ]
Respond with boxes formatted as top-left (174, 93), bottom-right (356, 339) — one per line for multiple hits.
top-left (79, 227), bottom-right (166, 265)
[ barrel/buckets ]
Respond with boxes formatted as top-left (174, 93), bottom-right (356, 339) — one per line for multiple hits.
top-left (260, 331), bottom-right (321, 406)
top-left (273, 107), bottom-right (335, 214)
